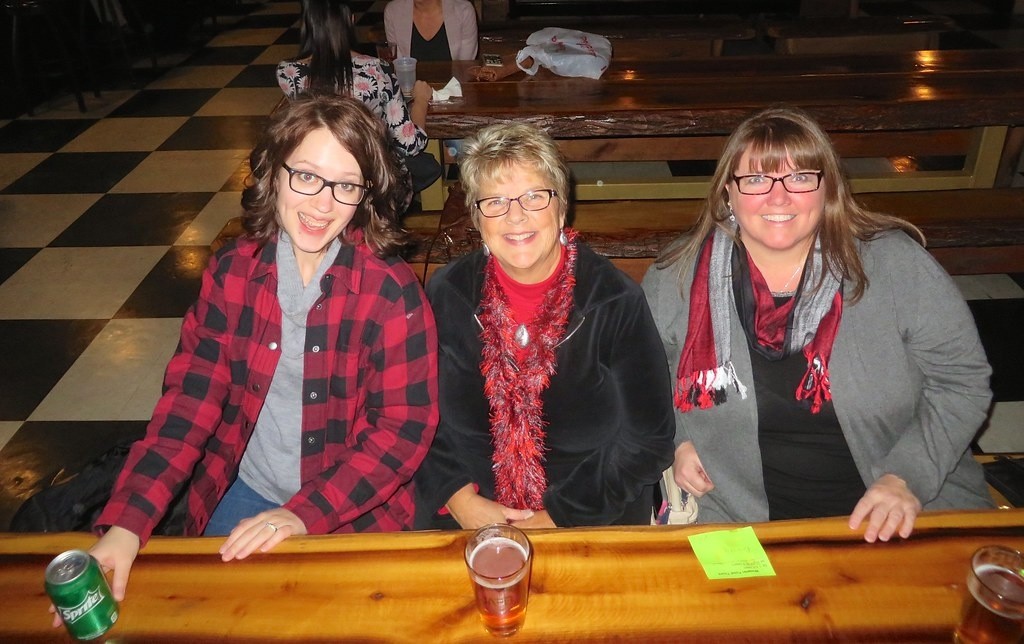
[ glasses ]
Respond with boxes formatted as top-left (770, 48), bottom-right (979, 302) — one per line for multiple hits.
top-left (274, 157), bottom-right (371, 205)
top-left (730, 169), bottom-right (824, 195)
top-left (473, 187), bottom-right (560, 217)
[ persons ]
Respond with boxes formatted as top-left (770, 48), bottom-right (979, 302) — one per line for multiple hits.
top-left (384, 0), bottom-right (479, 62)
top-left (638, 100), bottom-right (994, 543)
top-left (275, 0), bottom-right (433, 221)
top-left (411, 118), bottom-right (676, 532)
top-left (47, 85), bottom-right (441, 629)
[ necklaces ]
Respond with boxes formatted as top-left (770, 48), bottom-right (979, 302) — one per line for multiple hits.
top-left (750, 256), bottom-right (806, 293)
top-left (510, 317), bottom-right (535, 349)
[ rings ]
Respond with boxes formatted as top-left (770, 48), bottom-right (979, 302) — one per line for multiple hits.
top-left (266, 523), bottom-right (278, 532)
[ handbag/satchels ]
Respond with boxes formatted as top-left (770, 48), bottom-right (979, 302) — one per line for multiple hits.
top-left (516, 27), bottom-right (612, 78)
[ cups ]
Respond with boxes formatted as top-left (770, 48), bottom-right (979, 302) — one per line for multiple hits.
top-left (376, 42), bottom-right (397, 67)
top-left (952, 544), bottom-right (1024, 644)
top-left (394, 58), bottom-right (417, 97)
top-left (464, 524), bottom-right (532, 637)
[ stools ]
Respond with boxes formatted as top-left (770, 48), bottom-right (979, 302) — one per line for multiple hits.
top-left (6, 3), bottom-right (101, 115)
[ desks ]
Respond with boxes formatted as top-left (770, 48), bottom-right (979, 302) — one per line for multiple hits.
top-left (0, 508), bottom-right (1023, 640)
top-left (244, 0), bottom-right (1023, 294)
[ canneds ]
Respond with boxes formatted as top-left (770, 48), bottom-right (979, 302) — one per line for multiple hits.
top-left (43, 549), bottom-right (119, 642)
